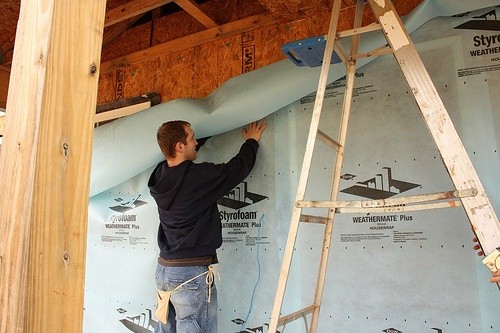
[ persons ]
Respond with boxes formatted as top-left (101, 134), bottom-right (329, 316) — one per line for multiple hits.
top-left (473, 238), bottom-right (500, 283)
top-left (147, 117), bottom-right (269, 333)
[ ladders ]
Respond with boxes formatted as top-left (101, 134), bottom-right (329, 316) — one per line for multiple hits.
top-left (265, 1), bottom-right (499, 333)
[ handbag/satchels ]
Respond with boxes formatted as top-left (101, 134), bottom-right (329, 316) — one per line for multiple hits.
top-left (155, 265), bottom-right (220, 326)
